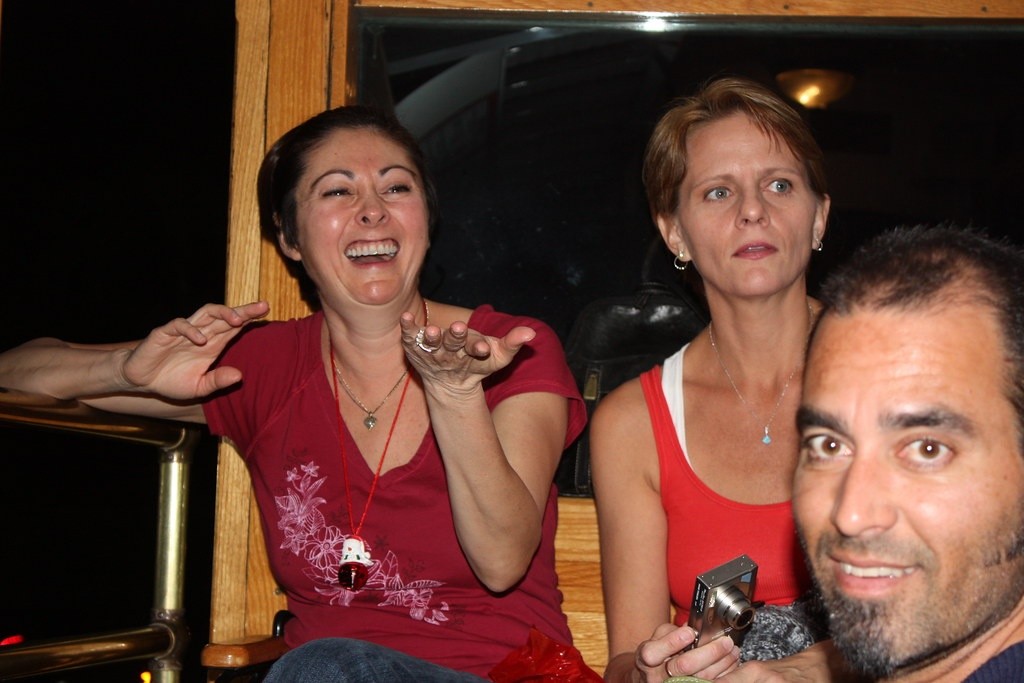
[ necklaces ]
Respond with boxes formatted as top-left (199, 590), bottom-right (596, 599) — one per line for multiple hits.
top-left (707, 297), bottom-right (816, 443)
top-left (329, 299), bottom-right (429, 428)
top-left (329, 293), bottom-right (428, 591)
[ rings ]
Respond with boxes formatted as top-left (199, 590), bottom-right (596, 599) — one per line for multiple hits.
top-left (415, 328), bottom-right (443, 352)
top-left (665, 657), bottom-right (674, 677)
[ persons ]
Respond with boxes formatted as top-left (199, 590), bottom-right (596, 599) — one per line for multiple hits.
top-left (0, 104), bottom-right (609, 683)
top-left (790, 215), bottom-right (1024, 683)
top-left (588, 75), bottom-right (834, 683)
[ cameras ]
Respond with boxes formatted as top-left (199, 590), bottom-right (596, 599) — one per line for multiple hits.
top-left (684, 554), bottom-right (758, 653)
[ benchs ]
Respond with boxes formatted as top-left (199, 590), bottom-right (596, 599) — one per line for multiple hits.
top-left (203, 498), bottom-right (611, 683)
